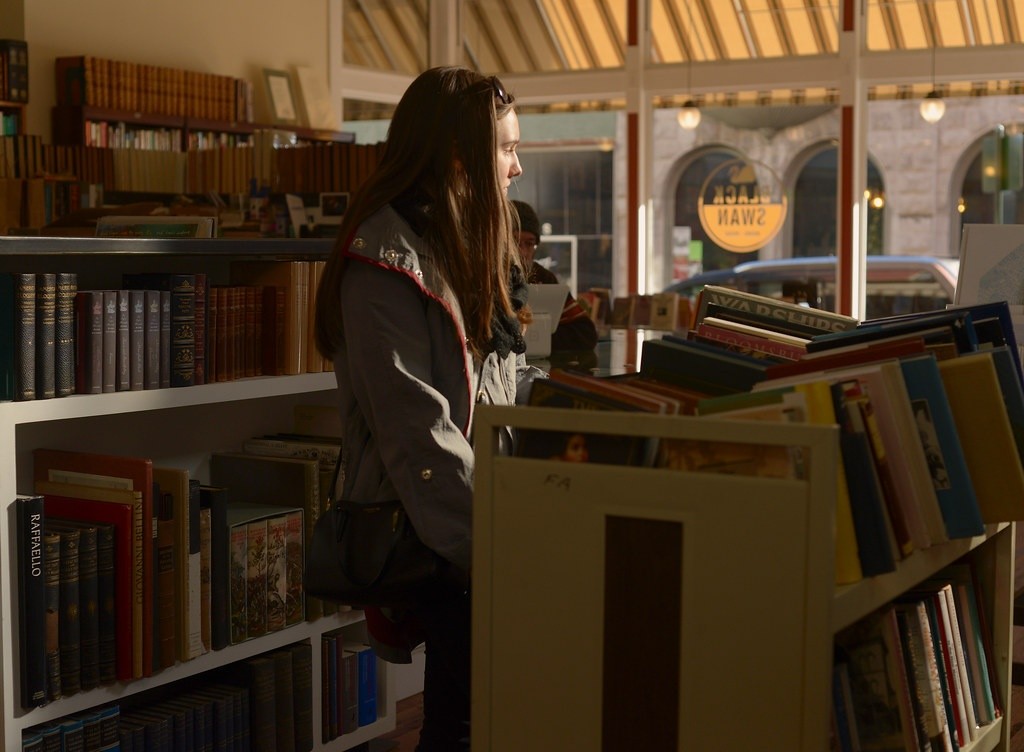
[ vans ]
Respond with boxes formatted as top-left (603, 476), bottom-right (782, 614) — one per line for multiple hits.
top-left (661, 256), bottom-right (959, 319)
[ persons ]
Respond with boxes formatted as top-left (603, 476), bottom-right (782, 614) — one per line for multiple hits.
top-left (313, 65), bottom-right (549, 752)
top-left (507, 197), bottom-right (599, 376)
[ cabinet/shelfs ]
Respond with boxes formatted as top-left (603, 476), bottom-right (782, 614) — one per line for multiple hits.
top-left (0, 224), bottom-right (399, 752)
top-left (462, 402), bottom-right (1024, 752)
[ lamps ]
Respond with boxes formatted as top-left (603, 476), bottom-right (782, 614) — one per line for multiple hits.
top-left (676, 48), bottom-right (702, 132)
top-left (919, 21), bottom-right (944, 125)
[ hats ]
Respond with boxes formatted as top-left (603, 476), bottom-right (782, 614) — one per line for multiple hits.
top-left (510, 200), bottom-right (541, 245)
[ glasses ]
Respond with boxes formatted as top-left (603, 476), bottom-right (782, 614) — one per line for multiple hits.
top-left (458, 75), bottom-right (508, 104)
top-left (520, 238), bottom-right (538, 250)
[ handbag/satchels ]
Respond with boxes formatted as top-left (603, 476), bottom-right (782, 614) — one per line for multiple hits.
top-left (302, 499), bottom-right (432, 609)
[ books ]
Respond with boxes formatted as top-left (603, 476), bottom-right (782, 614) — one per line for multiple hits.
top-left (9, 408), bottom-right (380, 752)
top-left (1, 40), bottom-right (387, 231)
top-left (8, 261), bottom-right (341, 400)
top-left (506, 283), bottom-right (1023, 751)
